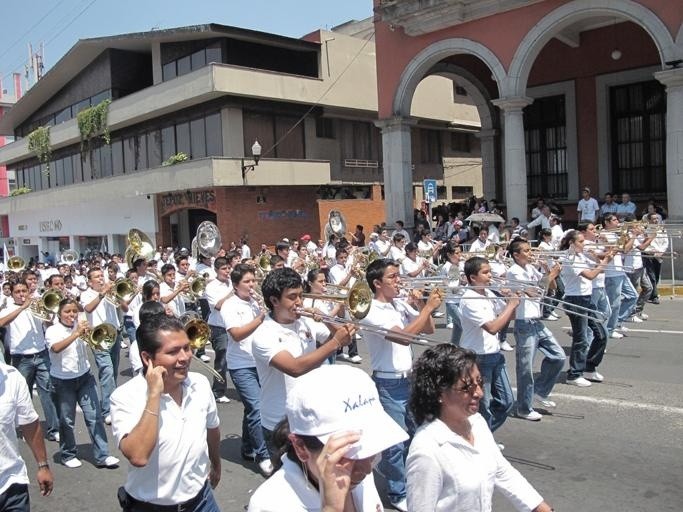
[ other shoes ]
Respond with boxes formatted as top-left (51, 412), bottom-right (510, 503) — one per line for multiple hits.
top-left (205, 341), bottom-right (209, 344)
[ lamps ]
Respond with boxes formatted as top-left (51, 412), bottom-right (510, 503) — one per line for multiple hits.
top-left (241, 136), bottom-right (260, 180)
top-left (609, 16), bottom-right (622, 61)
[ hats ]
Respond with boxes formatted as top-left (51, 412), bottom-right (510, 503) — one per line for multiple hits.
top-left (282, 237), bottom-right (289, 243)
top-left (180, 247), bottom-right (187, 251)
top-left (300, 234), bottom-right (312, 240)
top-left (285, 364), bottom-right (412, 460)
top-left (369, 232), bottom-right (379, 240)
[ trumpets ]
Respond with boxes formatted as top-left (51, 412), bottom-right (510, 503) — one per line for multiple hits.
top-left (98, 279), bottom-right (137, 308)
top-left (252, 249), bottom-right (377, 284)
top-left (7, 256), bottom-right (26, 272)
top-left (60, 248), bottom-right (79, 266)
top-left (71, 320), bottom-right (118, 354)
top-left (165, 310), bottom-right (223, 382)
top-left (27, 288), bottom-right (63, 322)
top-left (171, 275), bottom-right (208, 300)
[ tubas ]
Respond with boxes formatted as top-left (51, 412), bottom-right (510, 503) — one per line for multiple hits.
top-left (125, 228), bottom-right (155, 270)
top-left (323, 209), bottom-right (347, 243)
top-left (191, 220), bottom-right (222, 260)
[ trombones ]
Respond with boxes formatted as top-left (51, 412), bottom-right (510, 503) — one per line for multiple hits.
top-left (526, 223), bottom-right (681, 272)
top-left (250, 285), bottom-right (372, 319)
top-left (294, 305), bottom-right (457, 350)
top-left (393, 246), bottom-right (605, 321)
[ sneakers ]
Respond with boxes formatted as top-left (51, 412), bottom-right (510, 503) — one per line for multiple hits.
top-left (446, 322), bottom-right (452, 328)
top-left (623, 314), bottom-right (643, 323)
top-left (241, 450), bottom-right (273, 478)
top-left (603, 349), bottom-right (607, 353)
top-left (532, 392), bottom-right (556, 409)
top-left (199, 355), bottom-right (212, 363)
top-left (543, 311), bottom-right (560, 320)
top-left (582, 371), bottom-right (604, 382)
top-left (500, 341), bottom-right (513, 352)
top-left (609, 326), bottom-right (628, 339)
top-left (94, 456), bottom-right (121, 467)
top-left (647, 297), bottom-right (660, 304)
top-left (60, 457), bottom-right (82, 468)
top-left (337, 352), bottom-right (363, 363)
top-left (47, 432), bottom-right (60, 442)
top-left (413, 338), bottom-right (427, 344)
top-left (215, 395), bottom-right (232, 404)
top-left (104, 415), bottom-right (112, 425)
top-left (636, 312), bottom-right (648, 319)
top-left (433, 312), bottom-right (443, 317)
top-left (356, 334), bottom-right (361, 338)
top-left (388, 495), bottom-right (407, 511)
top-left (121, 341), bottom-right (127, 349)
top-left (565, 376), bottom-right (592, 387)
top-left (516, 407), bottom-right (544, 420)
top-left (562, 327), bottom-right (572, 335)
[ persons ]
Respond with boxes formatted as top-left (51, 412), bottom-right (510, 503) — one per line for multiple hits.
top-left (1, 186), bottom-right (671, 512)
